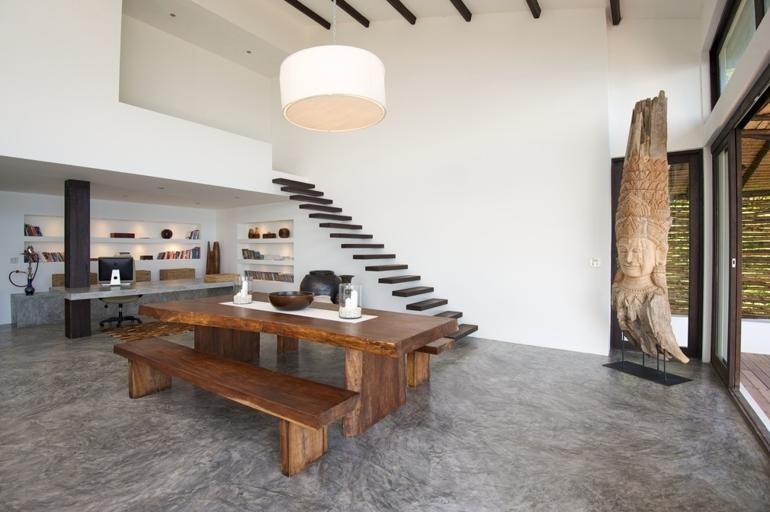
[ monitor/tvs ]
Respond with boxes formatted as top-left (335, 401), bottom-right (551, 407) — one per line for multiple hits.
top-left (97, 257), bottom-right (136, 286)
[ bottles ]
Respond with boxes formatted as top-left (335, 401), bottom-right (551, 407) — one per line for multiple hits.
top-left (337, 274), bottom-right (354, 301)
top-left (278, 228), bottom-right (290, 238)
top-left (160, 229), bottom-right (172, 239)
top-left (300, 270), bottom-right (341, 304)
top-left (206, 241), bottom-right (213, 275)
top-left (249, 225), bottom-right (260, 238)
top-left (212, 241), bottom-right (220, 273)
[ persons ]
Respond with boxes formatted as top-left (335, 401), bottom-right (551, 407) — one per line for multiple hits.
top-left (157, 229), bottom-right (200, 260)
top-left (614, 237), bottom-right (667, 294)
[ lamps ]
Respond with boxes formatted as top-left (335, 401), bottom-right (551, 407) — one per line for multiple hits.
top-left (279, 0), bottom-right (388, 134)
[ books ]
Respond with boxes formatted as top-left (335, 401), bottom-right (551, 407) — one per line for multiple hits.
top-left (23, 223), bottom-right (66, 263)
top-left (242, 248), bottom-right (294, 282)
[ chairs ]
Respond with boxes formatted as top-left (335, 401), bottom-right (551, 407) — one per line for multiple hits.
top-left (96, 254), bottom-right (143, 332)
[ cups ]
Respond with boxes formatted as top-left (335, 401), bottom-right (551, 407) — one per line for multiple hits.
top-left (232, 276), bottom-right (253, 304)
top-left (338, 282), bottom-right (362, 320)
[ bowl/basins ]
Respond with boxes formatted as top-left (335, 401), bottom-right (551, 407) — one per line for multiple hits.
top-left (269, 291), bottom-right (314, 310)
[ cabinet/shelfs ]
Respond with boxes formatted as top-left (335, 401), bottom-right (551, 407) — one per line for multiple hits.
top-left (236, 219), bottom-right (296, 282)
top-left (23, 214), bottom-right (202, 263)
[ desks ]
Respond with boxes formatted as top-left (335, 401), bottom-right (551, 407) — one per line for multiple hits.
top-left (48, 279), bottom-right (235, 339)
top-left (137, 288), bottom-right (458, 439)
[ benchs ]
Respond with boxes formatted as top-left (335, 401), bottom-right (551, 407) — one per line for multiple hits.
top-left (113, 336), bottom-right (361, 478)
top-left (275, 331), bottom-right (456, 389)
